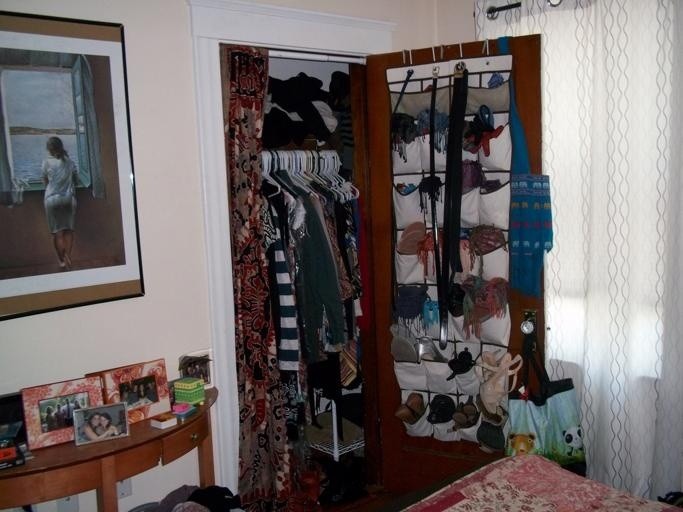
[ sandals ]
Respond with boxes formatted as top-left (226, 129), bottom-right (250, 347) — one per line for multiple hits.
top-left (451, 395), bottom-right (479, 432)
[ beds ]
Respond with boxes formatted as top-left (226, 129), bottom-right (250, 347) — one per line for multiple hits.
top-left (400, 452), bottom-right (682, 512)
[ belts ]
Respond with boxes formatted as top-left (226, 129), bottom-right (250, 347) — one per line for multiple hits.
top-left (438, 61), bottom-right (470, 349)
top-left (429, 66), bottom-right (440, 308)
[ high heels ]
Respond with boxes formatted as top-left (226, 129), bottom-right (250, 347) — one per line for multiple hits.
top-left (416, 336), bottom-right (443, 364)
top-left (476, 350), bottom-right (523, 414)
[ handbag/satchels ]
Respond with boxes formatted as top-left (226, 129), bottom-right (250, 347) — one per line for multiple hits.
top-left (504, 339), bottom-right (587, 478)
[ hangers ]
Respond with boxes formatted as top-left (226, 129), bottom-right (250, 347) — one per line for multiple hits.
top-left (260, 150), bottom-right (360, 204)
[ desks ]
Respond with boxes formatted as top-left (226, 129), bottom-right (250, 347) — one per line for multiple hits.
top-left (0, 384), bottom-right (219, 512)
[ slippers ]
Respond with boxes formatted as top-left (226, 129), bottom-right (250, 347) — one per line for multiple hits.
top-left (398, 222), bottom-right (425, 253)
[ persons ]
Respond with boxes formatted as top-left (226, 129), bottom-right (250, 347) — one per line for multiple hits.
top-left (94, 414), bottom-right (119, 440)
top-left (40, 137), bottom-right (80, 269)
top-left (55, 404), bottom-right (64, 428)
top-left (62, 398), bottom-right (75, 425)
top-left (73, 401), bottom-right (80, 411)
top-left (80, 412), bottom-right (113, 441)
top-left (45, 406), bottom-right (54, 431)
top-left (187, 365), bottom-right (207, 382)
top-left (124, 382), bottom-right (156, 405)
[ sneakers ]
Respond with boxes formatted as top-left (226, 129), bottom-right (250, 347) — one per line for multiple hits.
top-left (394, 393), bottom-right (424, 425)
top-left (387, 322), bottom-right (417, 363)
top-left (426, 394), bottom-right (455, 424)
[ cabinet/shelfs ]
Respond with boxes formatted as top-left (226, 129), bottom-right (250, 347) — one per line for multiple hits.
top-left (218, 35), bottom-right (543, 496)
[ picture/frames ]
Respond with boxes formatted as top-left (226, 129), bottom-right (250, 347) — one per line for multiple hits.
top-left (1, 12), bottom-right (146, 323)
top-left (72, 403), bottom-right (132, 446)
top-left (21, 376), bottom-right (104, 451)
top-left (85, 357), bottom-right (173, 423)
